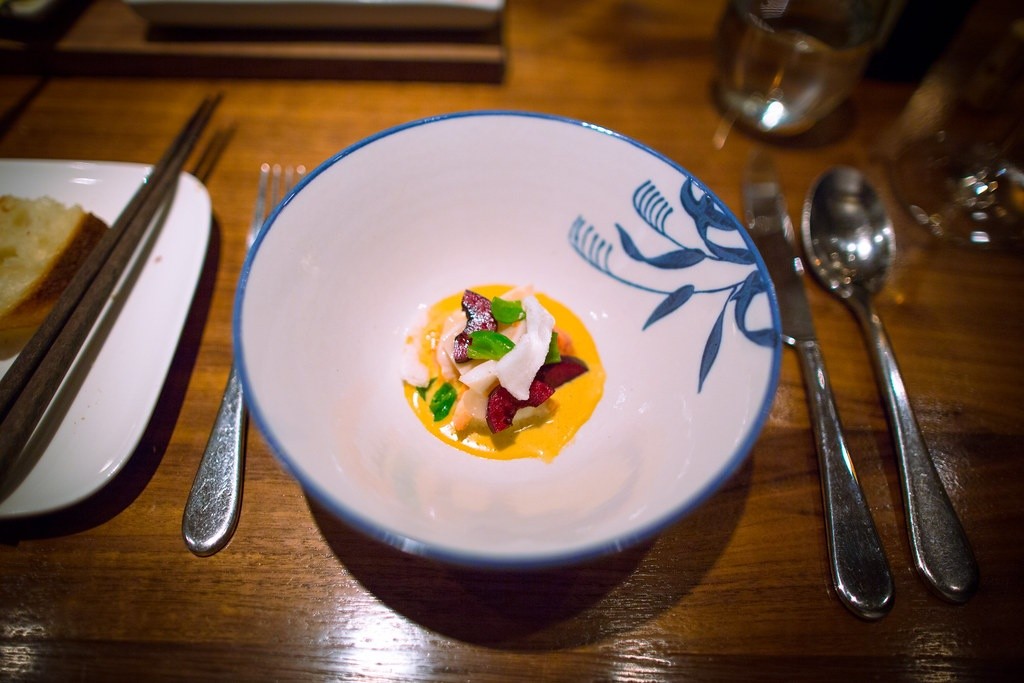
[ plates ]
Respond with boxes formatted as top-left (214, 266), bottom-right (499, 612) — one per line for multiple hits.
top-left (0, 157), bottom-right (212, 517)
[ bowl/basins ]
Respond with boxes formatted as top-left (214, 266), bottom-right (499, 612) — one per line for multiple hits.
top-left (234, 111), bottom-right (780, 568)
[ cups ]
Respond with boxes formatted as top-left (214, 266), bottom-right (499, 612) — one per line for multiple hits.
top-left (885, 0), bottom-right (1023, 247)
top-left (713, 0), bottom-right (888, 137)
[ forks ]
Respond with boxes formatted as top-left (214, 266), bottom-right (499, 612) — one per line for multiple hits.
top-left (182, 164), bottom-right (305, 556)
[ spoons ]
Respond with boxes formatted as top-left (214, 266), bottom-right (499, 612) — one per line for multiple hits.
top-left (802, 163), bottom-right (979, 604)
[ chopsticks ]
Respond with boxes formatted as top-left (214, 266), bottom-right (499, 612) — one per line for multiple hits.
top-left (0, 92), bottom-right (224, 477)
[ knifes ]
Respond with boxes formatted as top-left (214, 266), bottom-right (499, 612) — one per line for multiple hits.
top-left (743, 149), bottom-right (894, 620)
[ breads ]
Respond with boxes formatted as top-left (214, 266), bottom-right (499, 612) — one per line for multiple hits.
top-left (0, 194), bottom-right (111, 360)
